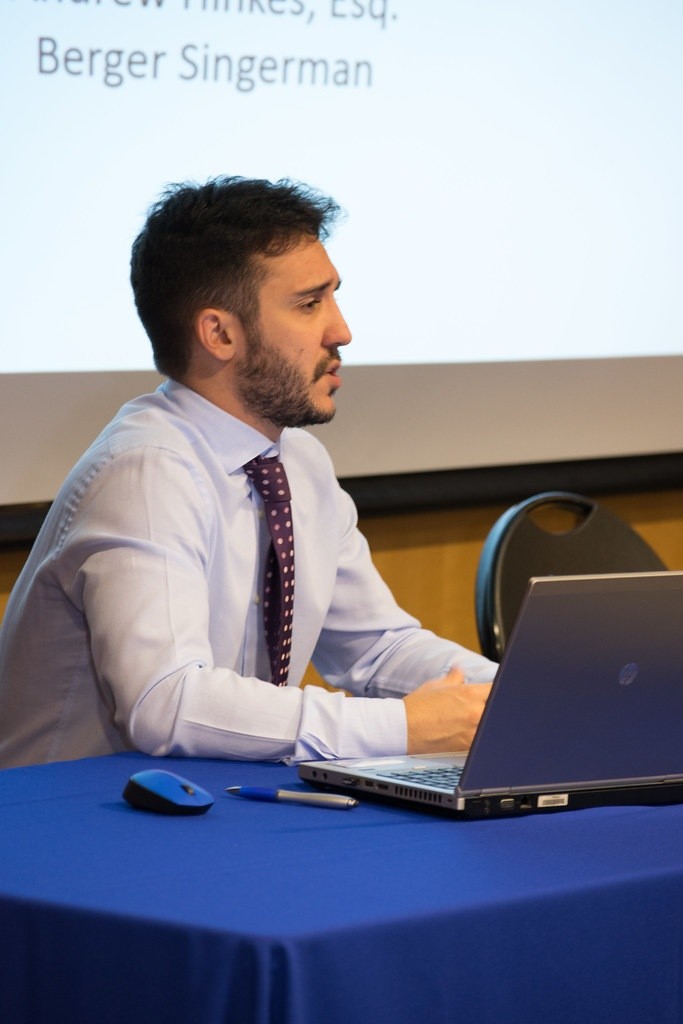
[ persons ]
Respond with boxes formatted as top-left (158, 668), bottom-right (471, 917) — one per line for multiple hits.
top-left (0, 178), bottom-right (505, 779)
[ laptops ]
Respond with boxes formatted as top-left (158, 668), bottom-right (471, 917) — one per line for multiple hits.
top-left (298, 570), bottom-right (683, 817)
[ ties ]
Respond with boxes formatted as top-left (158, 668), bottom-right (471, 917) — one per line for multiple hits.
top-left (242, 453), bottom-right (295, 687)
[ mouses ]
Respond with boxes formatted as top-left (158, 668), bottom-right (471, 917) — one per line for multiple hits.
top-left (121, 768), bottom-right (214, 816)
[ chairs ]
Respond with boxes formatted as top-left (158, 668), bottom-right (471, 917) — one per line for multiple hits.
top-left (475, 491), bottom-right (671, 663)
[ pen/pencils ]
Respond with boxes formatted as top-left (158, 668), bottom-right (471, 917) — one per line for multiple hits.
top-left (223, 785), bottom-right (360, 811)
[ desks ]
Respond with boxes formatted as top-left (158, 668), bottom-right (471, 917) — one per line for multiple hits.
top-left (1, 751), bottom-right (683, 1024)
